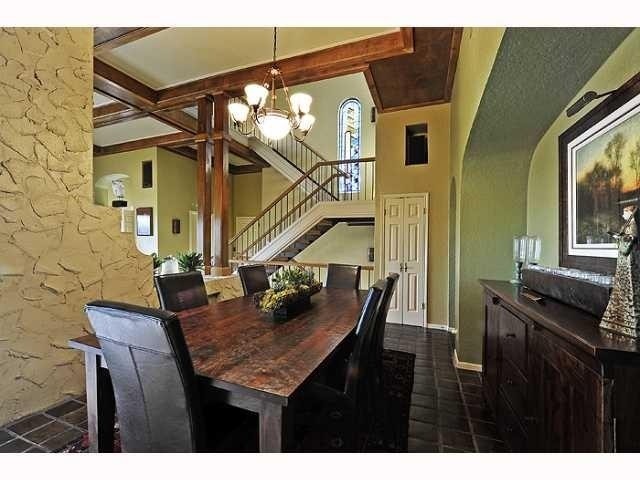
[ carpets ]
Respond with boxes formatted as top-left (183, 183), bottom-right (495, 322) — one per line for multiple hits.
top-left (0, 346), bottom-right (418, 453)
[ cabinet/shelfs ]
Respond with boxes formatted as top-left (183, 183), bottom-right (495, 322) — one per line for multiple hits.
top-left (475, 277), bottom-right (637, 450)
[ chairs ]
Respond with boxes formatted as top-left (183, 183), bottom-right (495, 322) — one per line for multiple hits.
top-left (305, 277), bottom-right (385, 453)
top-left (356, 273), bottom-right (398, 411)
top-left (84, 295), bottom-right (205, 453)
top-left (154, 272), bottom-right (209, 315)
top-left (238, 264), bottom-right (270, 296)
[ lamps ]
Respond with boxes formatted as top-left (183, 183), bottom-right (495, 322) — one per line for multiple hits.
top-left (227, 28), bottom-right (317, 143)
top-left (566, 89), bottom-right (615, 117)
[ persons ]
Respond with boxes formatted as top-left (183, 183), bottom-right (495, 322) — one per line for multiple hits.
top-left (598, 205), bottom-right (639, 339)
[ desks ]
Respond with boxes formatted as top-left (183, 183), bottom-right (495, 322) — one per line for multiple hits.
top-left (66, 283), bottom-right (379, 453)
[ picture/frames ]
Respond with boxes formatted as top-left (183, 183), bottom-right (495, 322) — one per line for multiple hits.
top-left (554, 68), bottom-right (640, 277)
